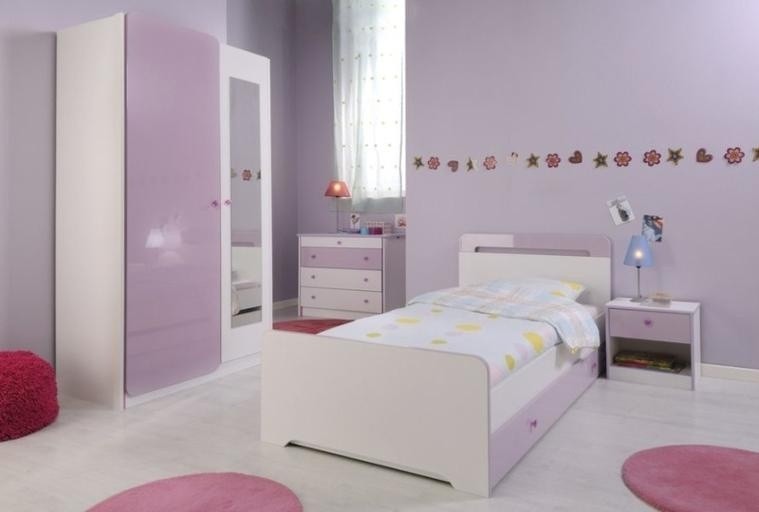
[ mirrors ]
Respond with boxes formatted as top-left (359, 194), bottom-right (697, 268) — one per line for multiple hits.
top-left (228, 75), bottom-right (264, 328)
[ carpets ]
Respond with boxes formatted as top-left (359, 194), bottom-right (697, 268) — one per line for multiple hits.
top-left (272, 319), bottom-right (353, 334)
top-left (87, 471), bottom-right (303, 512)
top-left (620, 443), bottom-right (759, 511)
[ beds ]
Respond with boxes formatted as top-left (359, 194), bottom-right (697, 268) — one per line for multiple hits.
top-left (260, 233), bottom-right (613, 498)
top-left (231, 231), bottom-right (262, 315)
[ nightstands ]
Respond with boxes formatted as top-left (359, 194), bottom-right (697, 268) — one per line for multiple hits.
top-left (604, 298), bottom-right (701, 391)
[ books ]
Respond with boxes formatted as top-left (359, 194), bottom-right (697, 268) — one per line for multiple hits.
top-left (615, 350), bottom-right (680, 368)
top-left (613, 359), bottom-right (689, 374)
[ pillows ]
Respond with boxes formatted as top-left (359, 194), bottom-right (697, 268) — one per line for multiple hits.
top-left (512, 278), bottom-right (586, 306)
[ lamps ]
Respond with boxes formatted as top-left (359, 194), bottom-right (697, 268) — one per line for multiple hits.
top-left (624, 235), bottom-right (654, 302)
top-left (324, 180), bottom-right (350, 232)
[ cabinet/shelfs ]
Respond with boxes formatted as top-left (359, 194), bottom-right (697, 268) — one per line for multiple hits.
top-left (54, 11), bottom-right (272, 412)
top-left (296, 234), bottom-right (405, 320)
